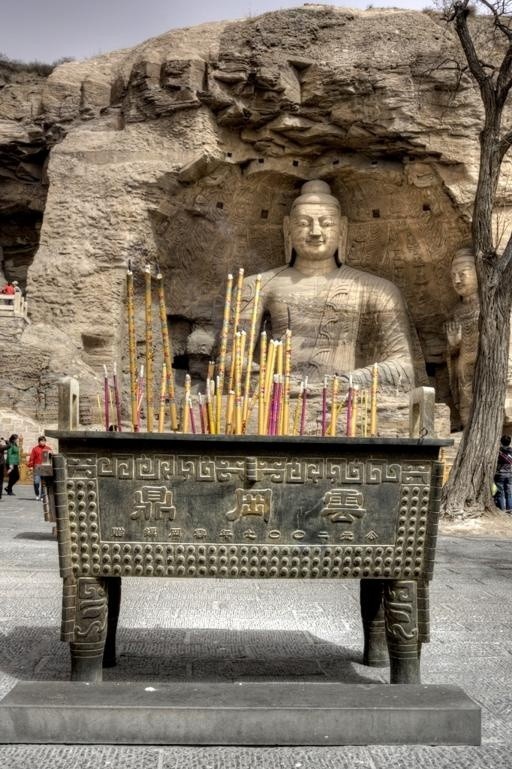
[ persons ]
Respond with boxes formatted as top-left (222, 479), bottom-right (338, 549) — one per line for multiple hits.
top-left (439, 246), bottom-right (508, 432)
top-left (11, 281), bottom-right (22, 297)
top-left (212, 181), bottom-right (424, 396)
top-left (0, 438), bottom-right (11, 499)
top-left (494, 434), bottom-right (512, 513)
top-left (29, 435), bottom-right (55, 501)
top-left (2, 281), bottom-right (15, 304)
top-left (5, 434), bottom-right (21, 495)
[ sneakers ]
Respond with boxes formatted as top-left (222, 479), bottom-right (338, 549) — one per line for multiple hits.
top-left (4, 487), bottom-right (15, 495)
top-left (36, 496), bottom-right (45, 501)
